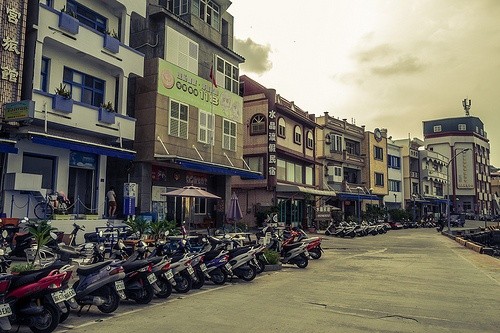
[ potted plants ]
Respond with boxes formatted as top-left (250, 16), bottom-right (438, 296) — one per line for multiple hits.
top-left (104, 29), bottom-right (120, 53)
top-left (52, 83), bottom-right (73, 114)
top-left (59, 5), bottom-right (79, 35)
top-left (98, 101), bottom-right (115, 124)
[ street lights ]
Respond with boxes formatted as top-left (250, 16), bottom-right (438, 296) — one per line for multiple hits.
top-left (446, 148), bottom-right (469, 232)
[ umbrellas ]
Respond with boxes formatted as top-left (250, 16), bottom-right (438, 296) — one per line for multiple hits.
top-left (160, 184), bottom-right (220, 236)
top-left (227, 192), bottom-right (243, 233)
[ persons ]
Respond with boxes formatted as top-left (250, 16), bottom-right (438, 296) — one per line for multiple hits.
top-left (106, 187), bottom-right (117, 217)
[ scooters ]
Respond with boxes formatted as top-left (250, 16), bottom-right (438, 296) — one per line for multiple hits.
top-left (265, 226), bottom-right (330, 269)
top-left (0, 216), bottom-right (265, 333)
top-left (324, 218), bottom-right (436, 238)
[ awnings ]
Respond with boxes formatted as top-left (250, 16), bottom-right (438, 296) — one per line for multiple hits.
top-left (406, 197), bottom-right (452, 208)
top-left (276, 182), bottom-right (336, 199)
top-left (336, 193), bottom-right (382, 200)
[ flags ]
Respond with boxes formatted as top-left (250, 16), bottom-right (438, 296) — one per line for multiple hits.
top-left (209, 63), bottom-right (217, 88)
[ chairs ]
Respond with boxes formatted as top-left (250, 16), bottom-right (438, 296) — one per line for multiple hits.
top-left (23, 242), bottom-right (99, 268)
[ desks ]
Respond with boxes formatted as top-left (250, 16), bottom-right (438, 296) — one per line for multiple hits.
top-left (227, 232), bottom-right (253, 247)
top-left (168, 235), bottom-right (199, 240)
top-left (121, 238), bottom-right (155, 260)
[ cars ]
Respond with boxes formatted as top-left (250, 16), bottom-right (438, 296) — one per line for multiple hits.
top-left (450, 215), bottom-right (465, 227)
top-left (460, 212), bottom-right (500, 222)
